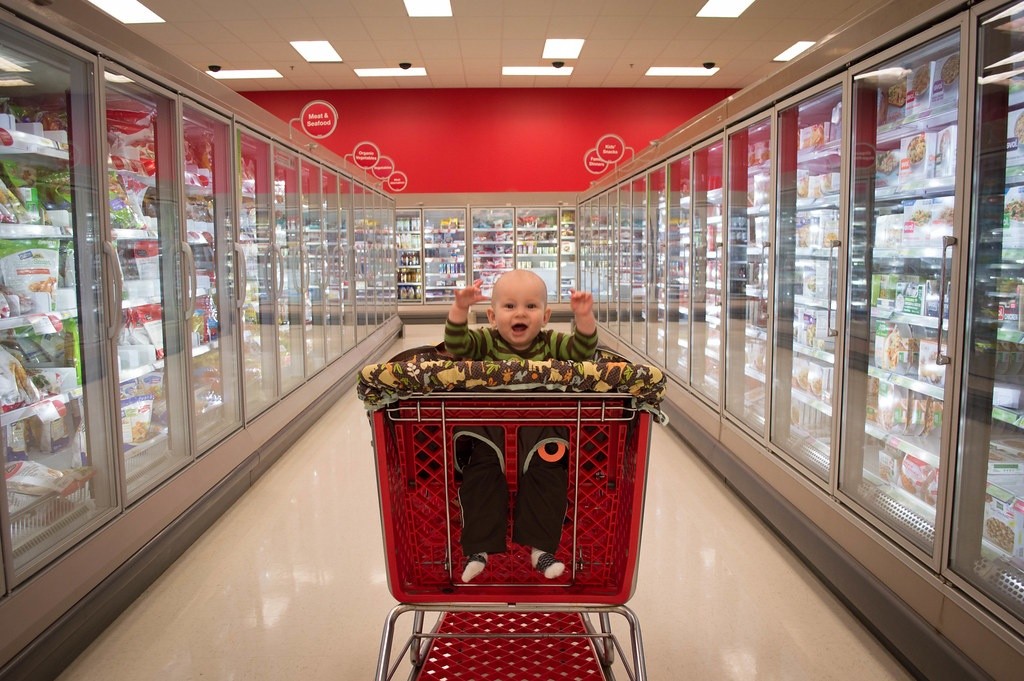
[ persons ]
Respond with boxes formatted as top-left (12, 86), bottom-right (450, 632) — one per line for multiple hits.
top-left (444, 269), bottom-right (598, 583)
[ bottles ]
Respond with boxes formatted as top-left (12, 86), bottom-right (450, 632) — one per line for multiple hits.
top-left (401, 252), bottom-right (419, 265)
top-left (399, 286), bottom-right (421, 299)
top-left (397, 268), bottom-right (421, 283)
top-left (396, 217), bottom-right (420, 231)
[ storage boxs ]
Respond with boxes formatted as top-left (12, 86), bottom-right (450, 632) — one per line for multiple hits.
top-left (795, 51), bottom-right (1024, 389)
top-left (877, 451), bottom-right (1023, 557)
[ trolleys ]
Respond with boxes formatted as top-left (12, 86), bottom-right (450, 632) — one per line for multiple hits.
top-left (359, 346), bottom-right (669, 681)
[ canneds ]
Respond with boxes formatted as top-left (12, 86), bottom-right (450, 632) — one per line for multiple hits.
top-left (517, 262), bottom-right (521, 269)
top-left (550, 261), bottom-right (556, 268)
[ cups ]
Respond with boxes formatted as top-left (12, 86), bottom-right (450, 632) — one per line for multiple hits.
top-left (518, 261), bottom-right (556, 268)
top-left (518, 245), bottom-right (556, 254)
